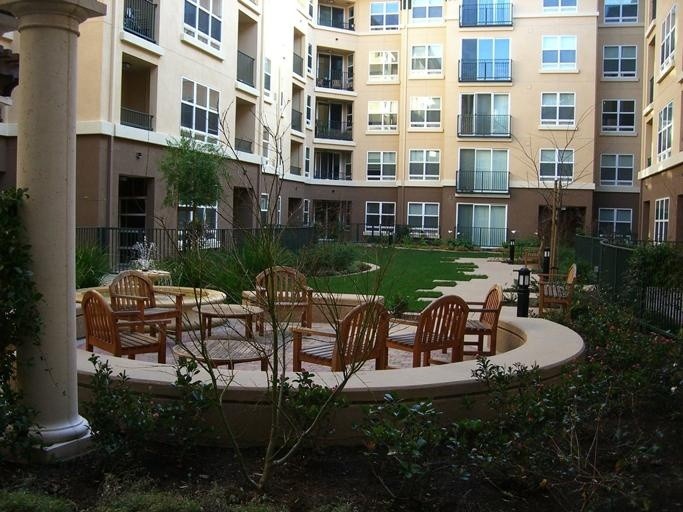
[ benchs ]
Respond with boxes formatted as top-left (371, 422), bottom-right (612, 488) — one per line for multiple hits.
top-left (537, 263), bottom-right (577, 317)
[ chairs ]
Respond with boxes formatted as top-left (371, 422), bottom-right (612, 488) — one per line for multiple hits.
top-left (81, 270), bottom-right (186, 364)
top-left (255, 265), bottom-right (314, 336)
top-left (289, 284), bottom-right (503, 372)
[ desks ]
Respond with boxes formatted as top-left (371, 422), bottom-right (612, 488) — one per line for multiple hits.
top-left (172, 340), bottom-right (275, 371)
top-left (191, 304), bottom-right (265, 339)
